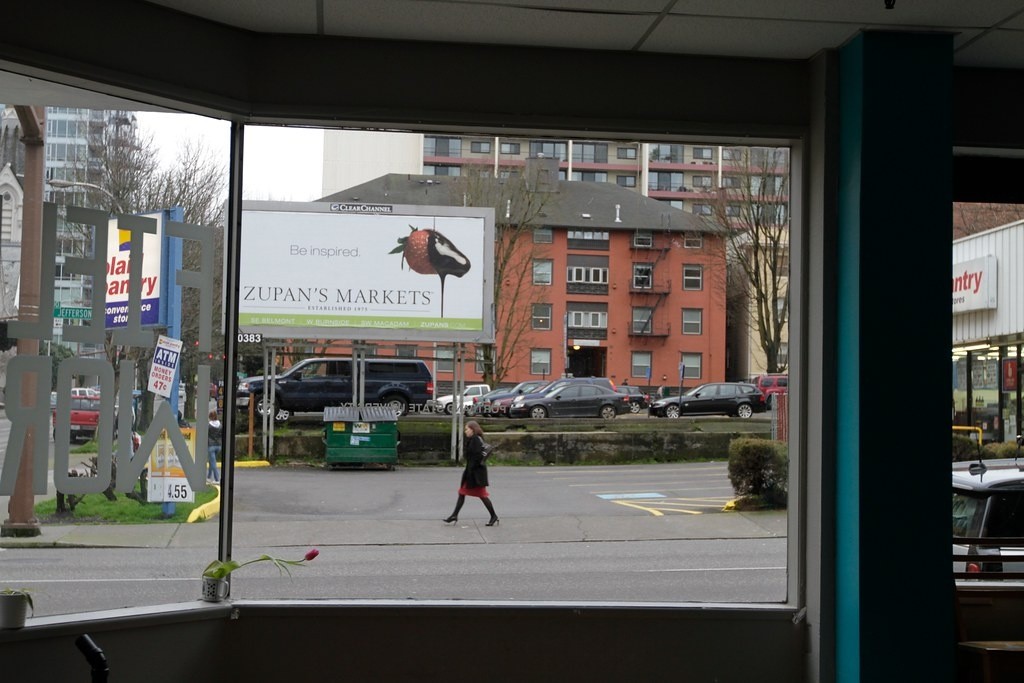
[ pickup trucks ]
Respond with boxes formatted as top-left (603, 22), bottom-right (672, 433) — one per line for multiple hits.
top-left (436, 385), bottom-right (491, 416)
top-left (52, 396), bottom-right (100, 441)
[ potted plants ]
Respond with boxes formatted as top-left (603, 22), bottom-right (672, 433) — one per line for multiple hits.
top-left (0, 586), bottom-right (50, 628)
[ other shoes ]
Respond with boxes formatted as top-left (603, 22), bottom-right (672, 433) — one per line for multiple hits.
top-left (206, 477), bottom-right (220, 485)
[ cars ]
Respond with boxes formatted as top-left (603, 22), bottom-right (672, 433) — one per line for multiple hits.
top-left (617, 386), bottom-right (650, 414)
top-left (952, 469), bottom-right (1023, 582)
top-left (510, 384), bottom-right (631, 419)
top-left (473, 381), bottom-right (545, 417)
top-left (491, 385), bottom-right (547, 416)
top-left (752, 376), bottom-right (787, 410)
top-left (650, 383), bottom-right (766, 418)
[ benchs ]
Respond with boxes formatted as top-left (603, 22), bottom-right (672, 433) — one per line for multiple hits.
top-left (951, 537), bottom-right (1024, 683)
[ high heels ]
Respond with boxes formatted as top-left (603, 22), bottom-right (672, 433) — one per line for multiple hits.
top-left (485, 517), bottom-right (499, 526)
top-left (441, 517), bottom-right (458, 525)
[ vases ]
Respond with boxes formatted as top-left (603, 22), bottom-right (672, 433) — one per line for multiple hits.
top-left (200, 576), bottom-right (229, 601)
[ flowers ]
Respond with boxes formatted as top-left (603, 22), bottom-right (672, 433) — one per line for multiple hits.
top-left (201, 548), bottom-right (320, 581)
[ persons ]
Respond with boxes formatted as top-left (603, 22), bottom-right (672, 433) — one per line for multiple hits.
top-left (621, 378), bottom-right (629, 386)
top-left (656, 385), bottom-right (663, 399)
top-left (609, 375), bottom-right (616, 387)
top-left (442, 420), bottom-right (500, 526)
top-left (178, 409), bottom-right (193, 428)
top-left (206, 410), bottom-right (222, 485)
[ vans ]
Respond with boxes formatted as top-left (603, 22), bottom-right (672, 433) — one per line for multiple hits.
top-left (236, 359), bottom-right (434, 423)
top-left (512, 378), bottom-right (618, 402)
